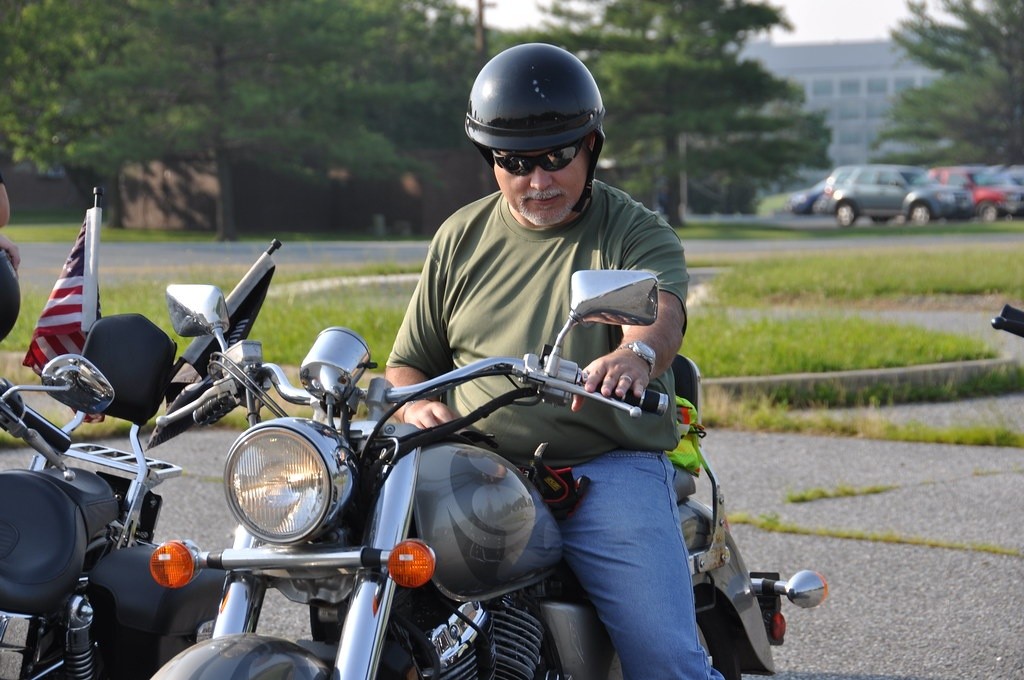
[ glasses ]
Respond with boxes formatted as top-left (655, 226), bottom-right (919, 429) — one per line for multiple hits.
top-left (495, 135), bottom-right (587, 178)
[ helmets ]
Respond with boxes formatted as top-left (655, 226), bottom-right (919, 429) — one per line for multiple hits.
top-left (466, 42), bottom-right (605, 174)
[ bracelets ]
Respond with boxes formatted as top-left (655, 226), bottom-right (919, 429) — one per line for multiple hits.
top-left (403, 397), bottom-right (432, 423)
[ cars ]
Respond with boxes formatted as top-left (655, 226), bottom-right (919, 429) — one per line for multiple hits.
top-left (790, 164), bottom-right (1024, 227)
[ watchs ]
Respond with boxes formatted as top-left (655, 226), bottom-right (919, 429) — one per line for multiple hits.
top-left (617, 341), bottom-right (656, 375)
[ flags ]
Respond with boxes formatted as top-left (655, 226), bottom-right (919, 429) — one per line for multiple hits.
top-left (23, 207), bottom-right (105, 421)
top-left (167, 255), bottom-right (276, 406)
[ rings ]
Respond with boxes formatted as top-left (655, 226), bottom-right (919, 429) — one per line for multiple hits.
top-left (620, 375), bottom-right (632, 382)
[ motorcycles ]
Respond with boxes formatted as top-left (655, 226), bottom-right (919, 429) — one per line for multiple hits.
top-left (0, 271), bottom-right (825, 680)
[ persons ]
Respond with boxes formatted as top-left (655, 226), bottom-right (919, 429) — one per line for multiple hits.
top-left (387, 42), bottom-right (724, 680)
top-left (0, 173), bottom-right (19, 271)
